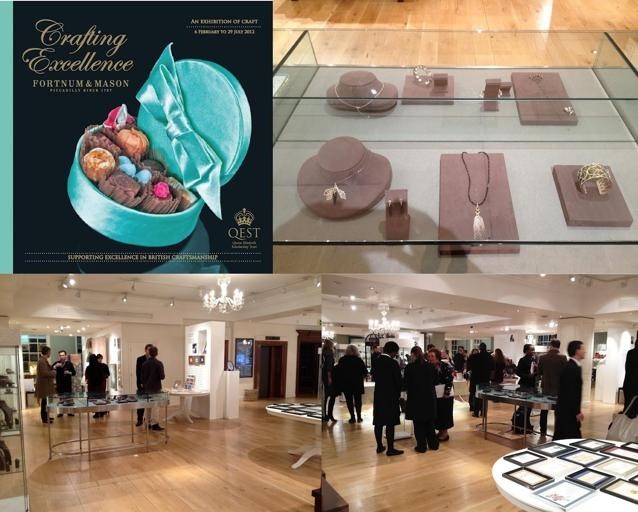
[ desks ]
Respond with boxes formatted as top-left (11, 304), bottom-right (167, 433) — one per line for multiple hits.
top-left (162, 386), bottom-right (210, 426)
top-left (476, 387), bottom-right (559, 449)
top-left (489, 437), bottom-right (637, 512)
top-left (44, 391), bottom-right (170, 466)
top-left (263, 401), bottom-right (322, 471)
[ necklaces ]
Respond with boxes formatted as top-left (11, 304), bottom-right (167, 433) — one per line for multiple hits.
top-left (458, 150), bottom-right (494, 242)
top-left (332, 82), bottom-right (386, 112)
top-left (310, 151), bottom-right (371, 206)
top-left (528, 72), bottom-right (575, 119)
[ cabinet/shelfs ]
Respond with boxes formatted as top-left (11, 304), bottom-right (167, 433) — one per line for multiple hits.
top-left (0, 346), bottom-right (30, 512)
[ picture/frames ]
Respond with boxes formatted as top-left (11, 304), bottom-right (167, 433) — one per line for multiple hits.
top-left (500, 438), bottom-right (637, 512)
top-left (234, 338), bottom-right (253, 378)
top-left (266, 401), bottom-right (323, 419)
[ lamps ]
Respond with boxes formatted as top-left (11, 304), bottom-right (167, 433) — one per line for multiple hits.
top-left (405, 306), bottom-right (435, 314)
top-left (199, 273), bottom-right (247, 316)
top-left (542, 319), bottom-right (559, 329)
top-left (58, 274), bottom-right (181, 310)
top-left (367, 302), bottom-right (400, 338)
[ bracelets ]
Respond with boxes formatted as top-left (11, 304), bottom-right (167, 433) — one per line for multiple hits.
top-left (574, 161), bottom-right (614, 198)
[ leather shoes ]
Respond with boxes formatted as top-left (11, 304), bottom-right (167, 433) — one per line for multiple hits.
top-left (377, 446), bottom-right (385, 453)
top-left (387, 449), bottom-right (404, 455)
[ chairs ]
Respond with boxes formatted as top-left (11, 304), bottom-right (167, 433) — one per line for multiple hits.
top-left (23, 375), bottom-right (39, 408)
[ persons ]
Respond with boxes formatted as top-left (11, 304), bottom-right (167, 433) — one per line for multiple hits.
top-left (31, 336), bottom-right (166, 435)
